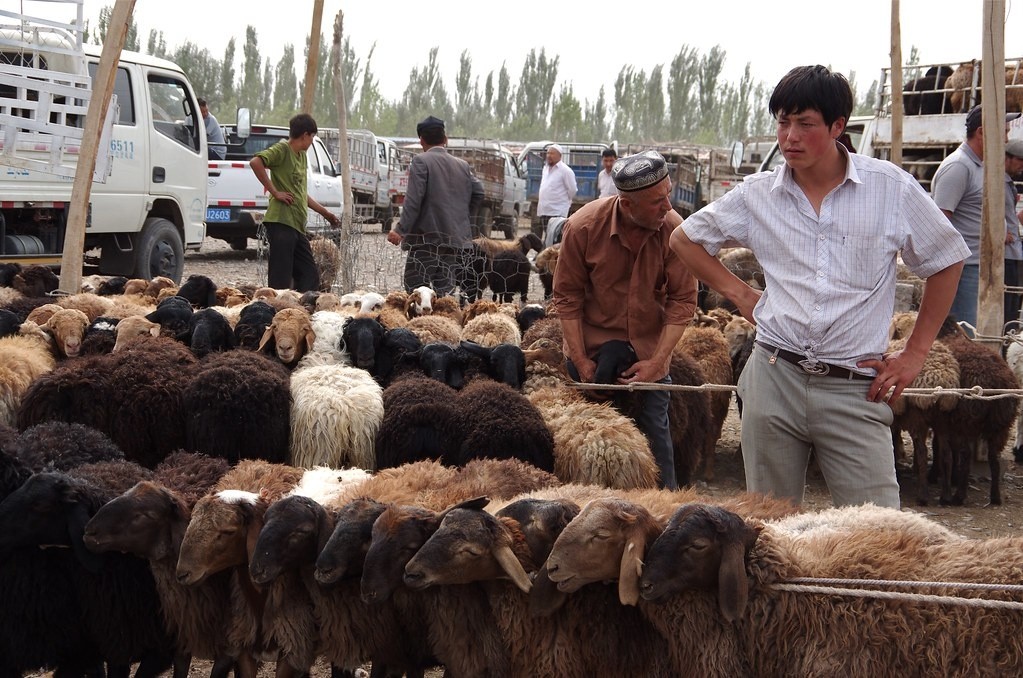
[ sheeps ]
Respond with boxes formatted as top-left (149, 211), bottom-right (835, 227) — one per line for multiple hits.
top-left (1, 232), bottom-right (1023, 678)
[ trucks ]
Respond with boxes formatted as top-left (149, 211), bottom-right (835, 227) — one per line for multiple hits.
top-left (757, 63), bottom-right (1023, 241)
top-left (706, 135), bottom-right (780, 205)
top-left (514, 140), bottom-right (620, 241)
top-left (625, 143), bottom-right (704, 221)
top-left (0, 0), bottom-right (252, 297)
top-left (388, 136), bottom-right (528, 239)
top-left (316, 128), bottom-right (405, 234)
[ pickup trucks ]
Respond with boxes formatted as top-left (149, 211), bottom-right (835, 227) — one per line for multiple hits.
top-left (205, 122), bottom-right (354, 252)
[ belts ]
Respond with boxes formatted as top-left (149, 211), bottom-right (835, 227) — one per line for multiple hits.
top-left (755, 339), bottom-right (875, 381)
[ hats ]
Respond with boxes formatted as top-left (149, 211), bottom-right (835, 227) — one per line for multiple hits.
top-left (610, 150), bottom-right (668, 192)
top-left (550, 143), bottom-right (563, 156)
top-left (417, 115), bottom-right (445, 138)
top-left (965, 103), bottom-right (1021, 131)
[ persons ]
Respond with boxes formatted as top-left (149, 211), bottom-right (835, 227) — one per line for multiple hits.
top-left (598, 149), bottom-right (619, 198)
top-left (1005, 138), bottom-right (1023, 334)
top-left (931, 104), bottom-right (1021, 339)
top-left (670, 64), bottom-right (971, 512)
top-left (536, 144), bottom-right (579, 235)
top-left (387, 116), bottom-right (485, 299)
top-left (250, 113), bottom-right (340, 293)
top-left (552, 149), bottom-right (697, 491)
top-left (184, 98), bottom-right (227, 160)
